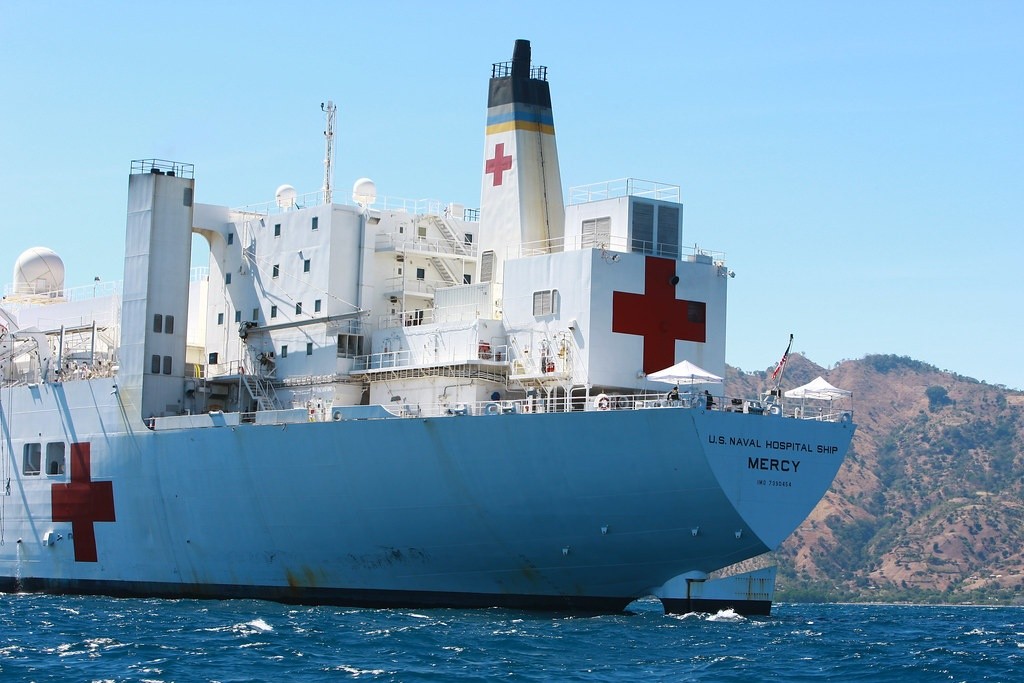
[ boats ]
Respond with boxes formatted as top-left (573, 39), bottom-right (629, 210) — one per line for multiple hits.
top-left (0, 39), bottom-right (857, 615)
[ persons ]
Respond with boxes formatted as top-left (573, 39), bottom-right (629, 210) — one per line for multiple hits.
top-left (54, 361), bottom-right (102, 382)
top-left (820, 407), bottom-right (823, 416)
top-left (704, 389), bottom-right (713, 409)
top-left (667, 387), bottom-right (685, 406)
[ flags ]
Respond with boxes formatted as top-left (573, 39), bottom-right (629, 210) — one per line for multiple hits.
top-left (772, 344), bottom-right (790, 381)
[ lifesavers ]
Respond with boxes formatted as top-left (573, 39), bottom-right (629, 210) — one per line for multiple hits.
top-left (598, 397), bottom-right (610, 410)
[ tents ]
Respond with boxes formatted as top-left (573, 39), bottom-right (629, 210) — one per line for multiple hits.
top-left (645, 360), bottom-right (724, 411)
top-left (784, 376), bottom-right (853, 420)
